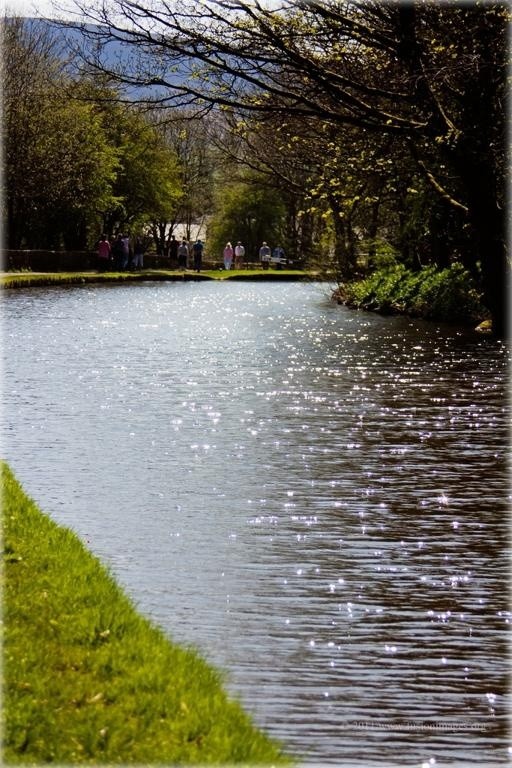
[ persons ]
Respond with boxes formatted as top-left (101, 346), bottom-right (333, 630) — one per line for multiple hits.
top-left (272, 243), bottom-right (286, 270)
top-left (169, 234), bottom-right (203, 273)
top-left (93, 233), bottom-right (145, 273)
top-left (223, 242), bottom-right (233, 270)
top-left (234, 241), bottom-right (245, 269)
top-left (259, 242), bottom-right (271, 269)
